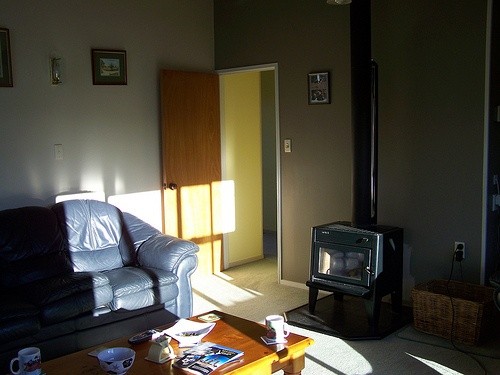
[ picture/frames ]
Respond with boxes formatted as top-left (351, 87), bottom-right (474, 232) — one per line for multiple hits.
top-left (0, 28), bottom-right (13, 87)
top-left (307, 70), bottom-right (330, 105)
top-left (91, 48), bottom-right (127, 85)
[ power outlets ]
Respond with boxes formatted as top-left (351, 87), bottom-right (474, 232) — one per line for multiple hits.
top-left (455, 241), bottom-right (465, 260)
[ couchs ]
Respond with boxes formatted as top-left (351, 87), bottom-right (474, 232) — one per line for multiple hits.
top-left (0, 200), bottom-right (199, 375)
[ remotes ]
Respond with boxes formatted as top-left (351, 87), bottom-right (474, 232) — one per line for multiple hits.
top-left (128, 329), bottom-right (158, 345)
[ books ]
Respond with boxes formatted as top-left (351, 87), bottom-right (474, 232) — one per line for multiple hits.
top-left (171, 341), bottom-right (244, 375)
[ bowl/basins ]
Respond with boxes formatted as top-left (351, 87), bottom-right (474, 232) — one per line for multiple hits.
top-left (98, 347), bottom-right (136, 375)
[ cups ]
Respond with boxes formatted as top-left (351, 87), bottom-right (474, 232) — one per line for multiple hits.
top-left (266, 314), bottom-right (290, 343)
top-left (9, 348), bottom-right (41, 375)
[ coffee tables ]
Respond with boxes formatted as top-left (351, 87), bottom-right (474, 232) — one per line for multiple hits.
top-left (42, 310), bottom-right (315, 375)
